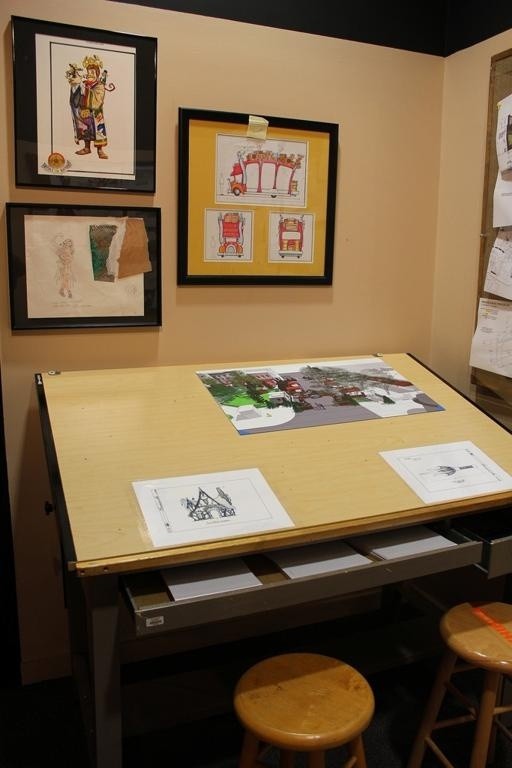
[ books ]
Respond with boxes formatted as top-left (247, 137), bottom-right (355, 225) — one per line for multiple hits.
top-left (354, 522), bottom-right (459, 560)
top-left (158, 557), bottom-right (263, 604)
top-left (265, 539), bottom-right (375, 579)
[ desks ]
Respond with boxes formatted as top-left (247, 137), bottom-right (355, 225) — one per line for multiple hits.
top-left (32, 347), bottom-right (512, 768)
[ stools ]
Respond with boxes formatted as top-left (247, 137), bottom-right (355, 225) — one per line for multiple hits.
top-left (229, 653), bottom-right (372, 766)
top-left (413, 598), bottom-right (511, 768)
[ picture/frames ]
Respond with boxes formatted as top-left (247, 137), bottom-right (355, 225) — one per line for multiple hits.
top-left (175, 104), bottom-right (341, 290)
top-left (5, 199), bottom-right (165, 332)
top-left (8, 12), bottom-right (159, 196)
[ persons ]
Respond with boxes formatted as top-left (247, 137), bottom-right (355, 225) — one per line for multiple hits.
top-left (63, 51), bottom-right (115, 158)
